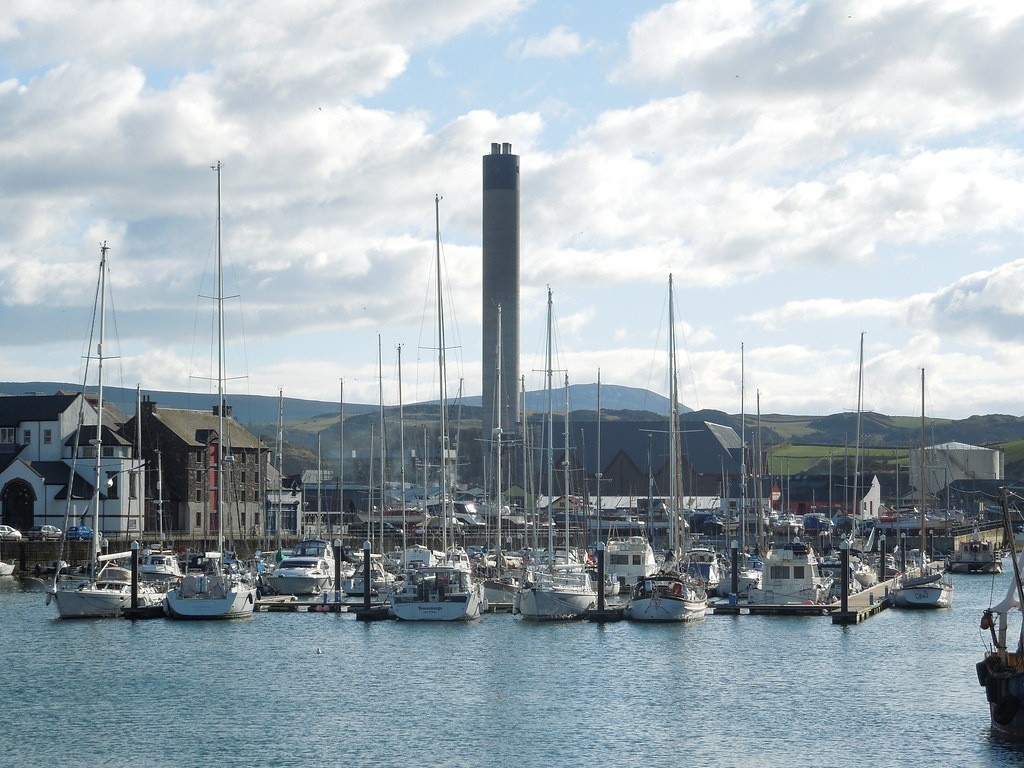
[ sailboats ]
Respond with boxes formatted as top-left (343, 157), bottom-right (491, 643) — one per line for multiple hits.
top-left (117, 332), bottom-right (929, 601)
top-left (45, 238), bottom-right (165, 617)
top-left (624, 274), bottom-right (708, 623)
top-left (519, 284), bottom-right (597, 620)
top-left (385, 192), bottom-right (486, 624)
top-left (167, 158), bottom-right (258, 620)
top-left (889, 366), bottom-right (954, 609)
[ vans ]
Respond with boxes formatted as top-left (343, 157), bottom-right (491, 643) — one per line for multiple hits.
top-left (0, 525), bottom-right (22, 541)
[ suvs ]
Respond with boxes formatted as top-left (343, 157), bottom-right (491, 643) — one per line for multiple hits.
top-left (28, 525), bottom-right (63, 542)
top-left (66, 526), bottom-right (93, 541)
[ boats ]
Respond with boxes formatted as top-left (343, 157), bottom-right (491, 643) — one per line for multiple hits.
top-left (977, 484), bottom-right (1024, 743)
top-left (0, 562), bottom-right (15, 575)
